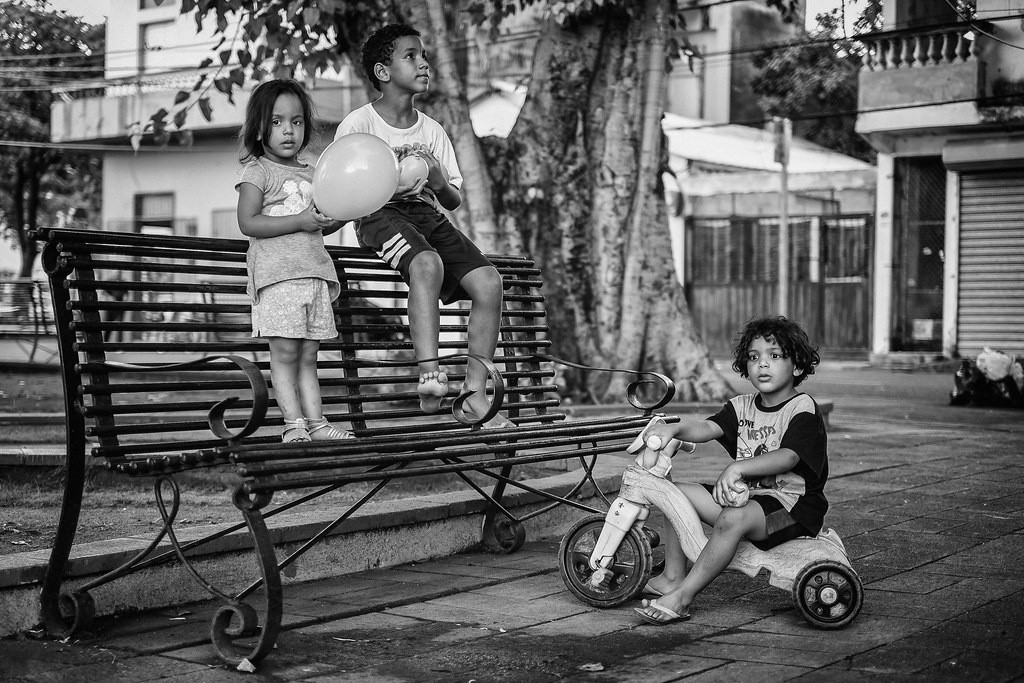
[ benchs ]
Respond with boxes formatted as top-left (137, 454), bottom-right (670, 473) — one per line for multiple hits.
top-left (42, 227), bottom-right (676, 669)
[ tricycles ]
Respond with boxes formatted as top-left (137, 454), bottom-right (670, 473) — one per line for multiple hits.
top-left (557, 413), bottom-right (867, 631)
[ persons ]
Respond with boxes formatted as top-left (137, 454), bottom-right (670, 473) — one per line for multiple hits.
top-left (333, 22), bottom-right (519, 430)
top-left (99, 269), bottom-right (130, 352)
top-left (630, 312), bottom-right (830, 626)
top-left (234, 76), bottom-right (403, 444)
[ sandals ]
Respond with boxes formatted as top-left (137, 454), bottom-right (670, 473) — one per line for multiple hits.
top-left (281, 419), bottom-right (312, 444)
top-left (306, 416), bottom-right (357, 442)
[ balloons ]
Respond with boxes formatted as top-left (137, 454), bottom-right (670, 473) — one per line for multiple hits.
top-left (396, 155), bottom-right (430, 191)
top-left (310, 130), bottom-right (400, 221)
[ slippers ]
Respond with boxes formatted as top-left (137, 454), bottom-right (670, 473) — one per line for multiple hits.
top-left (634, 579), bottom-right (675, 598)
top-left (631, 599), bottom-right (691, 626)
